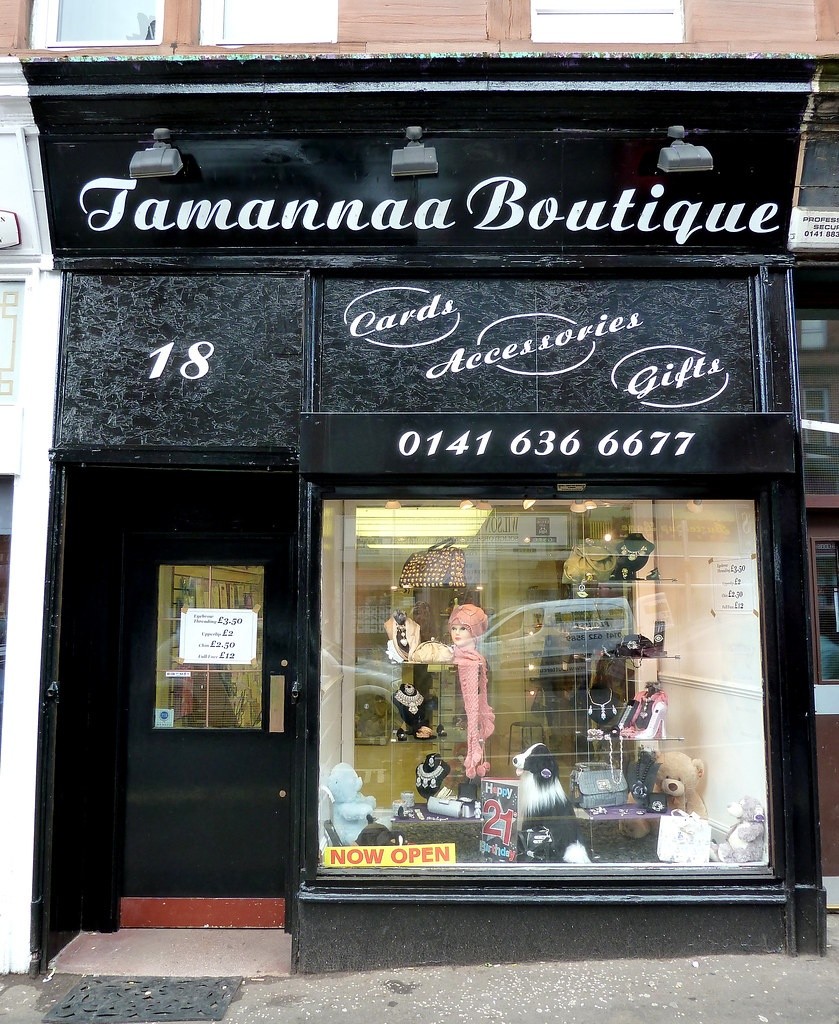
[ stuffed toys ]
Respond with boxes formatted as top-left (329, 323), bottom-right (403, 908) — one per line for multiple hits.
top-left (708, 795), bottom-right (767, 862)
top-left (323, 762), bottom-right (376, 846)
top-left (511, 742), bottom-right (592, 864)
top-left (618, 750), bottom-right (708, 839)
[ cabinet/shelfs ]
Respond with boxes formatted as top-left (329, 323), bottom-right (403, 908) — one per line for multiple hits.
top-left (573, 500), bottom-right (659, 763)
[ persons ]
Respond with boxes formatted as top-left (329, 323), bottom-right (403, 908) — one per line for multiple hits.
top-left (448, 603), bottom-right (487, 657)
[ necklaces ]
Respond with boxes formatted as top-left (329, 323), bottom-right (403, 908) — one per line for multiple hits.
top-left (395, 620), bottom-right (408, 647)
top-left (620, 540), bottom-right (650, 562)
top-left (638, 688), bottom-right (659, 720)
top-left (587, 686), bottom-right (618, 720)
top-left (607, 729), bottom-right (624, 784)
top-left (415, 760), bottom-right (445, 789)
top-left (393, 688), bottom-right (425, 715)
top-left (629, 753), bottom-right (657, 800)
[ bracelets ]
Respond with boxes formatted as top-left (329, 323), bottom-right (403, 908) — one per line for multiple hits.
top-left (435, 786), bottom-right (453, 799)
top-left (646, 799), bottom-right (665, 812)
top-left (416, 725), bottom-right (433, 739)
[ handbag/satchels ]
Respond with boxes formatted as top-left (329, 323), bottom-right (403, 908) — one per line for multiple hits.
top-left (570, 762), bottom-right (628, 808)
top-left (399, 537), bottom-right (468, 587)
top-left (412, 637), bottom-right (454, 664)
top-left (657, 809), bottom-right (712, 863)
top-left (615, 634), bottom-right (656, 669)
top-left (562, 545), bottom-right (616, 584)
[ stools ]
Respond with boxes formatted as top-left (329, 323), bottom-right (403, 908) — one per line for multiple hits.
top-left (507, 721), bottom-right (545, 765)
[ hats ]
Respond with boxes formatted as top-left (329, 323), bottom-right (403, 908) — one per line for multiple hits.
top-left (448, 604), bottom-right (488, 644)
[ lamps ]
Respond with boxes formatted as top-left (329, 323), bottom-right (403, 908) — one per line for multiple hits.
top-left (390, 126), bottom-right (439, 177)
top-left (686, 500), bottom-right (703, 513)
top-left (385, 499), bottom-right (401, 509)
top-left (570, 500), bottom-right (587, 513)
top-left (128, 128), bottom-right (183, 180)
top-left (475, 500), bottom-right (492, 510)
top-left (584, 499), bottom-right (598, 509)
top-left (657, 125), bottom-right (713, 173)
top-left (459, 500), bottom-right (473, 511)
